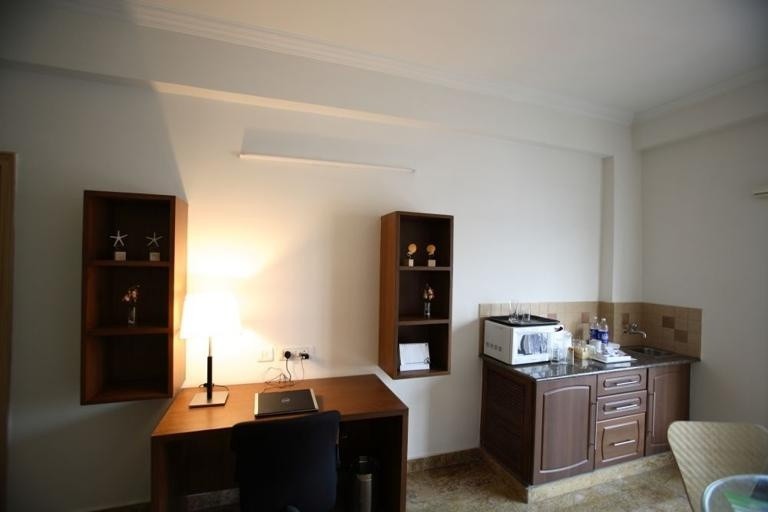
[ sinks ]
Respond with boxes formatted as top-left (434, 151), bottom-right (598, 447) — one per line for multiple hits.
top-left (631, 345), bottom-right (673, 359)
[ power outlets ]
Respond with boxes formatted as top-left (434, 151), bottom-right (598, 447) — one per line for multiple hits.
top-left (297, 345), bottom-right (314, 360)
top-left (282, 347), bottom-right (294, 360)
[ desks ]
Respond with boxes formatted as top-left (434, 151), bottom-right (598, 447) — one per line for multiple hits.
top-left (149, 374), bottom-right (411, 512)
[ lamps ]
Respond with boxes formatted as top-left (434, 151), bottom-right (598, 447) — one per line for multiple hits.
top-left (239, 153), bottom-right (416, 173)
top-left (188, 335), bottom-right (229, 407)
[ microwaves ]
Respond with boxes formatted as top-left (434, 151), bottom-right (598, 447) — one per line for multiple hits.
top-left (483, 320), bottom-right (563, 365)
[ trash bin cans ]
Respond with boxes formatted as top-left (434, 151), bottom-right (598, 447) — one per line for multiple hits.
top-left (345, 455), bottom-right (381, 511)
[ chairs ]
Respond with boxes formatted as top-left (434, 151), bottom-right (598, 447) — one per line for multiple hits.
top-left (232, 410), bottom-right (337, 512)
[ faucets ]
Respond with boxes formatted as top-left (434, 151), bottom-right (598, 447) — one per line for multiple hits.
top-left (626, 321), bottom-right (649, 340)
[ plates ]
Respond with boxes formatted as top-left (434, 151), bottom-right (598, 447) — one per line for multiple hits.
top-left (700, 473), bottom-right (768, 512)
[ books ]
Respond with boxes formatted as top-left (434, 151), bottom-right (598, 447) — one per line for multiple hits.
top-left (598, 349), bottom-right (632, 362)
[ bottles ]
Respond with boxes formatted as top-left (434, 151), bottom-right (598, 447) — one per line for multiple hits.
top-left (589, 316), bottom-right (598, 345)
top-left (598, 319), bottom-right (609, 355)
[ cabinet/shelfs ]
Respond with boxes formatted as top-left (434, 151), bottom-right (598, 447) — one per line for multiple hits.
top-left (79, 189), bottom-right (188, 407)
top-left (378, 208), bottom-right (455, 380)
top-left (480, 357), bottom-right (598, 486)
top-left (646, 363), bottom-right (693, 455)
top-left (595, 368), bottom-right (648, 469)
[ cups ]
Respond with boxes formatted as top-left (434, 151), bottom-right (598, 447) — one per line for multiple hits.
top-left (508, 300), bottom-right (531, 322)
